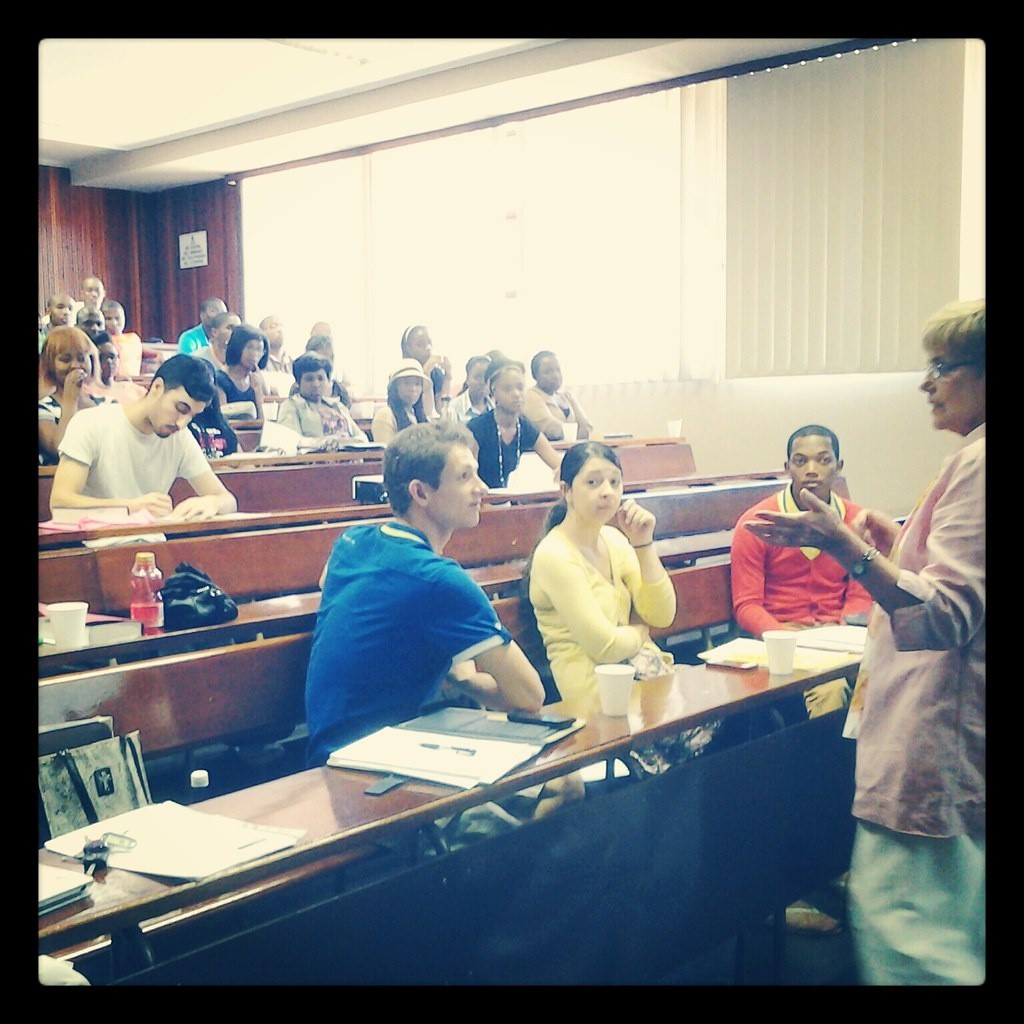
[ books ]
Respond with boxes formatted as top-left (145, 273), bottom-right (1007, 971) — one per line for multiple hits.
top-left (38, 602), bottom-right (141, 645)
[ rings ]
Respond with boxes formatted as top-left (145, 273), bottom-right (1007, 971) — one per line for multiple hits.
top-left (762, 530), bottom-right (772, 540)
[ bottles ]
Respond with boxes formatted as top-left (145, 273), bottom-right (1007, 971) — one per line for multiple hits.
top-left (184, 770), bottom-right (217, 802)
top-left (440, 395), bottom-right (451, 421)
top-left (131, 553), bottom-right (165, 635)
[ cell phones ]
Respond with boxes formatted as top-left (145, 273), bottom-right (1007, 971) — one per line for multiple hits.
top-left (507, 710), bottom-right (576, 730)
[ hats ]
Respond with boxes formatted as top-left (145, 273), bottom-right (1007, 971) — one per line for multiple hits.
top-left (386, 358), bottom-right (432, 392)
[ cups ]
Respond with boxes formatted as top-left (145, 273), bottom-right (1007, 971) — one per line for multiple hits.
top-left (562, 423), bottom-right (578, 441)
top-left (762, 630), bottom-right (800, 675)
top-left (666, 419), bottom-right (683, 438)
top-left (47, 601), bottom-right (89, 650)
top-left (594, 663), bottom-right (637, 716)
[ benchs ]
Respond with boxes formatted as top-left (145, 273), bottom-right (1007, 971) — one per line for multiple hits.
top-left (37, 343), bottom-right (913, 763)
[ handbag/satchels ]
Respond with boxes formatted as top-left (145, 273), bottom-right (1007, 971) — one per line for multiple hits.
top-left (159, 562), bottom-right (238, 628)
top-left (37, 730), bottom-right (152, 839)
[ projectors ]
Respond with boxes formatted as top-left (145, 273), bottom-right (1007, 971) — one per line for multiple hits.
top-left (352, 475), bottom-right (392, 504)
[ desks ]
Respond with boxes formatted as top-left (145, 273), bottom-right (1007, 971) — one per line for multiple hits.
top-left (37, 531), bottom-right (735, 676)
top-left (37, 468), bottom-right (784, 550)
top-left (200, 436), bottom-right (676, 473)
top-left (37, 626), bottom-right (867, 986)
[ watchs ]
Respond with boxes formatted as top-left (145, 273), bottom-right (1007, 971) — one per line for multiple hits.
top-left (849, 547), bottom-right (880, 579)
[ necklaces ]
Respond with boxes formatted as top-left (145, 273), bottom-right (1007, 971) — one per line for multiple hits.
top-left (494, 411), bottom-right (521, 488)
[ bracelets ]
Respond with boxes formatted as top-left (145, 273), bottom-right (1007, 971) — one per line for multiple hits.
top-left (633, 541), bottom-right (652, 548)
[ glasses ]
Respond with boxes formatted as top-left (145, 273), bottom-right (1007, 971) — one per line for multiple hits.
top-left (241, 346), bottom-right (267, 357)
top-left (926, 359), bottom-right (976, 380)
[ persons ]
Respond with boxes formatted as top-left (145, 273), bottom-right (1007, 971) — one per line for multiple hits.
top-left (305, 423), bottom-right (585, 820)
top-left (524, 441), bottom-right (677, 702)
top-left (731, 424), bottom-right (878, 718)
top-left (38, 277), bottom-right (592, 544)
top-left (743, 299), bottom-right (985, 987)
